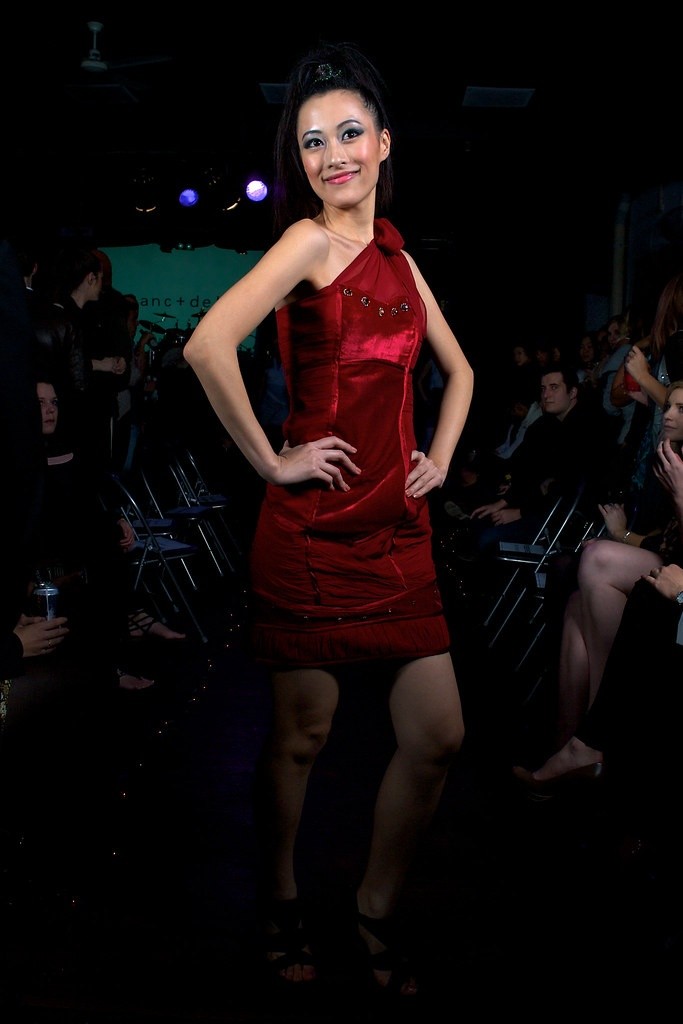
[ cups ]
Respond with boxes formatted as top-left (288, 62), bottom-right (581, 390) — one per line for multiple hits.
top-left (33, 588), bottom-right (61, 629)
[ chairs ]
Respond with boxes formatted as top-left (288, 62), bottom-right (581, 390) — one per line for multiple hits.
top-left (99, 444), bottom-right (241, 650)
top-left (480, 482), bottom-right (630, 707)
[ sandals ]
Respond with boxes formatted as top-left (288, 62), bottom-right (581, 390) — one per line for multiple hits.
top-left (261, 894), bottom-right (318, 986)
top-left (344, 888), bottom-right (426, 1001)
top-left (112, 664), bottom-right (158, 694)
top-left (125, 600), bottom-right (186, 647)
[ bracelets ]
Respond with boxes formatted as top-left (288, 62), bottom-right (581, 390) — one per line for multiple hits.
top-left (623, 531), bottom-right (633, 542)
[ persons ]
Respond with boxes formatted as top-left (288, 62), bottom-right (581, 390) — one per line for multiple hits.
top-left (468, 272), bottom-right (683, 793)
top-left (2, 229), bottom-right (187, 740)
top-left (184, 39), bottom-right (465, 998)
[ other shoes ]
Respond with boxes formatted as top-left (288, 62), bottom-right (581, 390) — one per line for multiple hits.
top-left (512, 745), bottom-right (615, 786)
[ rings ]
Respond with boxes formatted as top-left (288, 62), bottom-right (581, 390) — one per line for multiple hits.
top-left (48, 640), bottom-right (51, 648)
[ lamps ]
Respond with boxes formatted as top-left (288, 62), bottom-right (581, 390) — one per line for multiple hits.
top-left (246, 172), bottom-right (269, 201)
top-left (179, 178), bottom-right (200, 207)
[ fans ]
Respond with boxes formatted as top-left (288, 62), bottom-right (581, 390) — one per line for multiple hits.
top-left (49, 20), bottom-right (173, 91)
